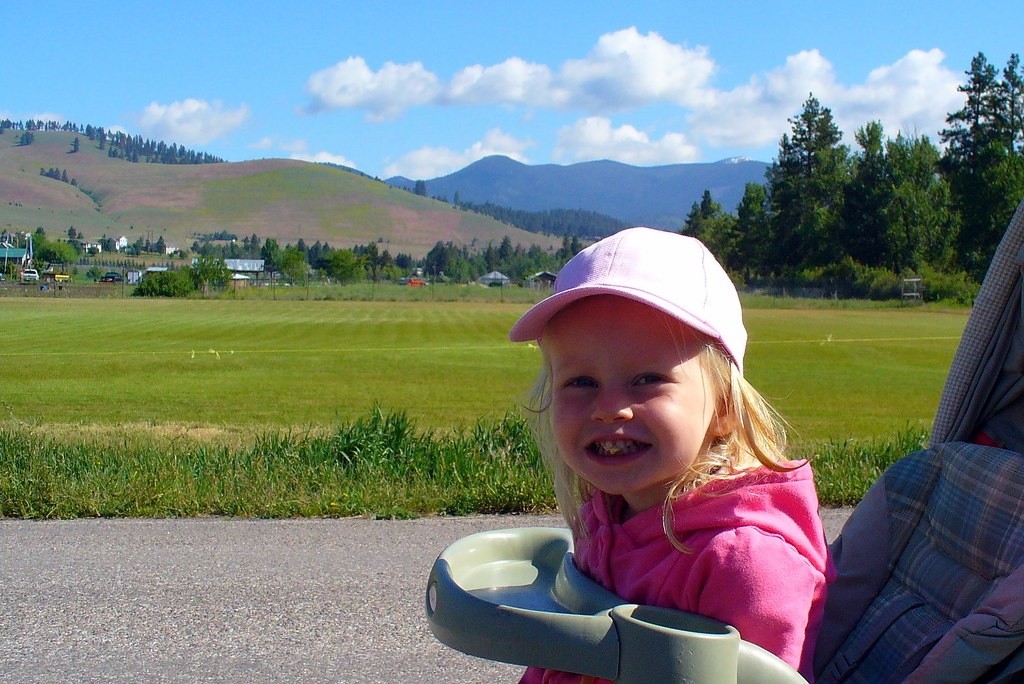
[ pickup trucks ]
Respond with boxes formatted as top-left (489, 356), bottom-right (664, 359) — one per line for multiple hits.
top-left (101, 272), bottom-right (128, 285)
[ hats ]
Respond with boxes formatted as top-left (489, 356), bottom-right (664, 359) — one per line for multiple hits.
top-left (510, 226), bottom-right (748, 377)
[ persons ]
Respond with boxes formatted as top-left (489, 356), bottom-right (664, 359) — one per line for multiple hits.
top-left (508, 227), bottom-right (839, 684)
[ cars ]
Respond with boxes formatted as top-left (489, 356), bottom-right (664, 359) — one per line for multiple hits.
top-left (22, 268), bottom-right (40, 282)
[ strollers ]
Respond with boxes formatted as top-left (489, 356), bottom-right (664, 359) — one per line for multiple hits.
top-left (423, 208), bottom-right (1024, 684)
